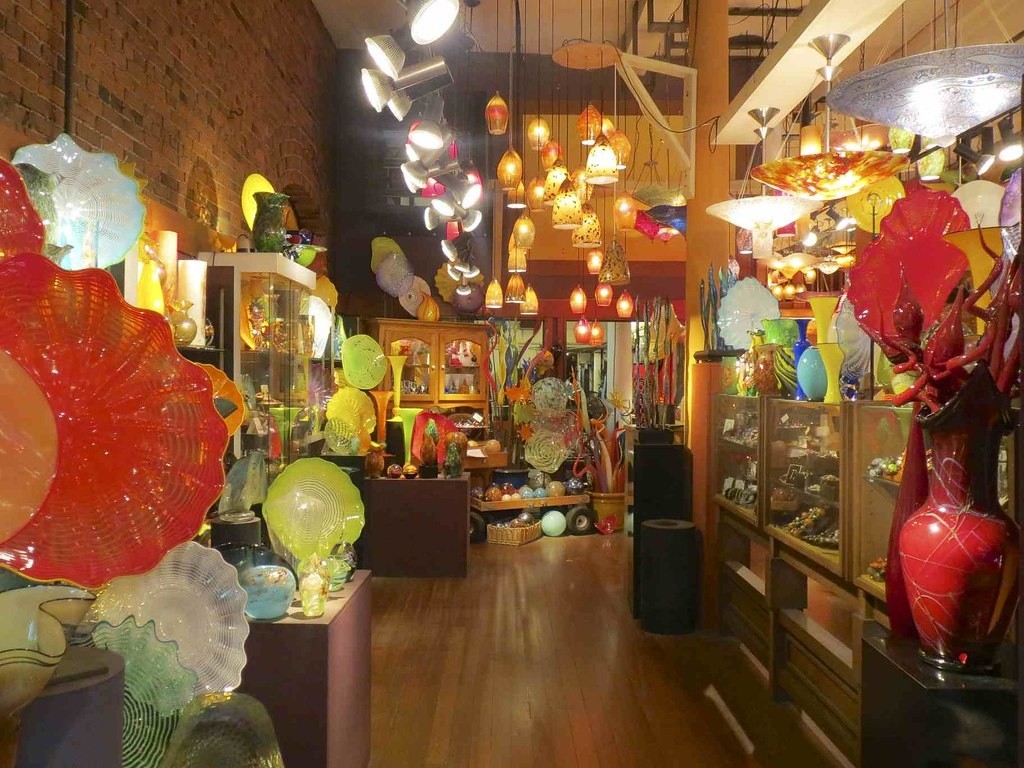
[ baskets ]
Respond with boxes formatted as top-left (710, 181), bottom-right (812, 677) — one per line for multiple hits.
top-left (486, 518), bottom-right (542, 547)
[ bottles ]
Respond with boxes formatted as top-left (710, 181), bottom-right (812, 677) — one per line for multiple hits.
top-left (252, 191), bottom-right (289, 252)
top-left (166, 300), bottom-right (197, 346)
top-left (720, 356), bottom-right (738, 394)
top-left (749, 294), bottom-right (843, 405)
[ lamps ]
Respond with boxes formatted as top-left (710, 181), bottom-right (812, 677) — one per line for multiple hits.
top-left (359, 0), bottom-right (1023, 349)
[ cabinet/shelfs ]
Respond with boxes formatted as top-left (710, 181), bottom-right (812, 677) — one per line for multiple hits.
top-left (366, 316), bottom-right (490, 440)
top-left (358, 473), bottom-right (471, 579)
top-left (758, 392), bottom-right (891, 579)
top-left (233, 568), bottom-right (372, 768)
top-left (707, 395), bottom-right (764, 529)
top-left (839, 399), bottom-right (1024, 601)
top-left (176, 251), bottom-right (341, 485)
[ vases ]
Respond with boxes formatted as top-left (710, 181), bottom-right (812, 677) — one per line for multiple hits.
top-left (582, 490), bottom-right (625, 533)
top-left (773, 348), bottom-right (799, 400)
top-left (251, 191), bottom-right (288, 253)
top-left (752, 342), bottom-right (783, 398)
top-left (898, 359), bottom-right (1023, 675)
top-left (792, 317), bottom-right (813, 401)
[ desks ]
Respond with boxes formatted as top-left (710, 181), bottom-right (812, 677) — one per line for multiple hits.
top-left (859, 635), bottom-right (1023, 767)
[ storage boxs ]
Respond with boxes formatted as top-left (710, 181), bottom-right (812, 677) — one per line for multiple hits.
top-left (486, 516), bottom-right (543, 548)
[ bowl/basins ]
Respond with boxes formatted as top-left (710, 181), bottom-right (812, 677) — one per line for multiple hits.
top-left (584, 490), bottom-right (628, 532)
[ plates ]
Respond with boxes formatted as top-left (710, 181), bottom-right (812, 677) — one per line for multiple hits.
top-left (524, 429), bottom-right (569, 473)
top-left (371, 236), bottom-right (431, 317)
top-left (0, 132), bottom-right (145, 272)
top-left (324, 417), bottom-right (357, 454)
top-left (0, 253), bottom-right (285, 768)
top-left (326, 387), bottom-right (377, 434)
top-left (340, 334), bottom-right (387, 389)
top-left (717, 276), bottom-right (780, 351)
top-left (261, 457), bottom-right (366, 561)
